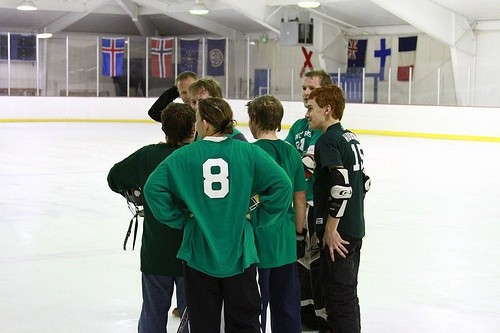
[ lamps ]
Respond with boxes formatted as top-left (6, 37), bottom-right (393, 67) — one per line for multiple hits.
top-left (188, 0), bottom-right (211, 16)
top-left (37, 26), bottom-right (53, 39)
top-left (16, 0), bottom-right (39, 11)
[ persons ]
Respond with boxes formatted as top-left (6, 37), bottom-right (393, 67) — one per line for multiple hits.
top-left (306, 84), bottom-right (372, 332)
top-left (285, 70), bottom-right (333, 333)
top-left (107, 70), bottom-right (309, 333)
